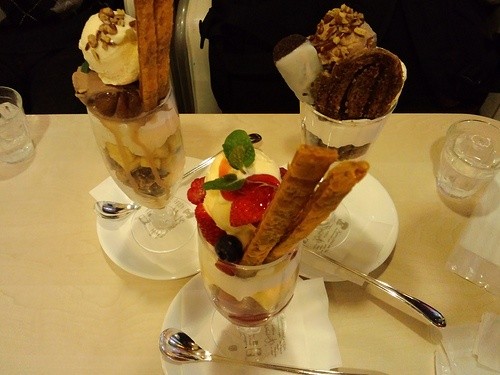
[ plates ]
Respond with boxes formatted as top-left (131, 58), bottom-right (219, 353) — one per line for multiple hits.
top-left (159, 270), bottom-right (331, 375)
top-left (282, 160), bottom-right (399, 283)
top-left (96, 157), bottom-right (203, 281)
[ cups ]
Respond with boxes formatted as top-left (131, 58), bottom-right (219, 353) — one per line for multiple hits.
top-left (437, 116), bottom-right (500, 217)
top-left (0, 85), bottom-right (34, 164)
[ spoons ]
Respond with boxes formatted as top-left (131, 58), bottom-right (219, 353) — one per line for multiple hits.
top-left (158, 326), bottom-right (369, 375)
top-left (94, 133), bottom-right (262, 219)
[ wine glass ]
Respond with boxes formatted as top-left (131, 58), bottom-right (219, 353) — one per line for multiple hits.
top-left (195, 216), bottom-right (300, 359)
top-left (300, 46), bottom-right (407, 252)
top-left (85, 74), bottom-right (198, 255)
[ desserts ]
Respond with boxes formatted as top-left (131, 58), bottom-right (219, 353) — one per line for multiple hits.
top-left (72, 0), bottom-right (182, 196)
top-left (274, 5), bottom-right (407, 159)
top-left (185, 133), bottom-right (369, 321)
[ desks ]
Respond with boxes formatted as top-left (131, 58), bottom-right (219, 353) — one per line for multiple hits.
top-left (1, 113), bottom-right (500, 375)
